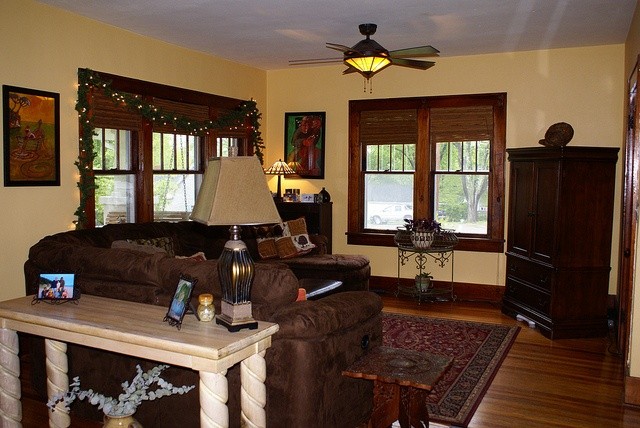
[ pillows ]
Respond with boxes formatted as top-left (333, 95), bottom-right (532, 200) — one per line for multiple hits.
top-left (175, 251), bottom-right (208, 261)
top-left (111, 241), bottom-right (168, 256)
top-left (251, 217), bottom-right (317, 258)
top-left (124, 237), bottom-right (176, 258)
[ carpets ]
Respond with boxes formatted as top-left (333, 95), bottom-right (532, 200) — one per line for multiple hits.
top-left (288, 215), bottom-right (371, 309)
top-left (381, 311), bottom-right (522, 426)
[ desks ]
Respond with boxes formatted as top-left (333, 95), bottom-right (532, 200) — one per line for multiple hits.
top-left (1, 292), bottom-right (279, 428)
top-left (393, 233), bottom-right (459, 304)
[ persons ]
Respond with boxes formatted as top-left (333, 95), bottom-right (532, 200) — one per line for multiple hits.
top-left (39, 276), bottom-right (69, 299)
top-left (169, 283), bottom-right (190, 321)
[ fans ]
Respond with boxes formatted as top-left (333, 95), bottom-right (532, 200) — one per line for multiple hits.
top-left (288, 24), bottom-right (439, 73)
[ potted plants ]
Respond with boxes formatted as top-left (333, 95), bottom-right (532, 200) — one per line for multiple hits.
top-left (404, 218), bottom-right (441, 247)
top-left (416, 272), bottom-right (431, 291)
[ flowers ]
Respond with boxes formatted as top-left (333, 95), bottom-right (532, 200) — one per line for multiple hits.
top-left (46, 362), bottom-right (196, 414)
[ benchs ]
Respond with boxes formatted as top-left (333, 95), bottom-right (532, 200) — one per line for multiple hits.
top-left (106, 211), bottom-right (190, 223)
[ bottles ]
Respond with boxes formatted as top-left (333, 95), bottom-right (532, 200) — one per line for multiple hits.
top-left (197, 294), bottom-right (215, 321)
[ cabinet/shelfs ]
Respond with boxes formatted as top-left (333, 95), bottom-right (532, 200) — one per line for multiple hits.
top-left (502, 147), bottom-right (621, 340)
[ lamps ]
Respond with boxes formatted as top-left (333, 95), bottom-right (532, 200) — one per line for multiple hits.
top-left (190, 153), bottom-right (284, 333)
top-left (343, 23), bottom-right (393, 80)
top-left (264, 158), bottom-right (295, 201)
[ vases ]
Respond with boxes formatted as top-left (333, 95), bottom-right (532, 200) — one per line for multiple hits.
top-left (101, 414), bottom-right (142, 428)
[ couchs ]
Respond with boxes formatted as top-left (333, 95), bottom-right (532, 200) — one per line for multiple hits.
top-left (24, 220), bottom-right (383, 428)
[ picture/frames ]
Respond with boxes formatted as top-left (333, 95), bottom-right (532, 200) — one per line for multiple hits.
top-left (163, 272), bottom-right (198, 330)
top-left (31, 267), bottom-right (81, 306)
top-left (2, 85), bottom-right (60, 187)
top-left (284, 111), bottom-right (326, 180)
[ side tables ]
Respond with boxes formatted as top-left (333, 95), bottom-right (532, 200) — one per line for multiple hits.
top-left (342, 344), bottom-right (455, 428)
